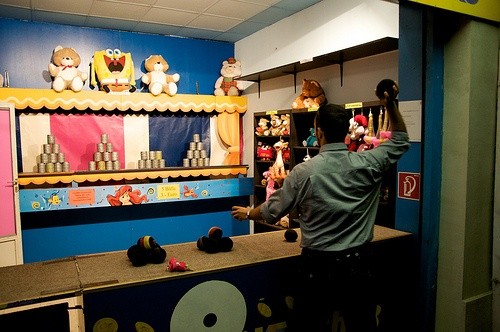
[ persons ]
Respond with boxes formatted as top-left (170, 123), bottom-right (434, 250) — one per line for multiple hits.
top-left (231, 81), bottom-right (411, 332)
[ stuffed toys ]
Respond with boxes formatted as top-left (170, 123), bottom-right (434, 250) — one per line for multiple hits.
top-left (254, 79), bottom-right (329, 228)
top-left (141, 54), bottom-right (180, 97)
top-left (214, 58), bottom-right (241, 96)
top-left (48, 46), bottom-right (137, 94)
top-left (343, 107), bottom-right (388, 153)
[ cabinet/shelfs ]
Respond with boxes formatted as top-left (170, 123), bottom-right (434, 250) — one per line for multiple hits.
top-left (254, 99), bottom-right (399, 234)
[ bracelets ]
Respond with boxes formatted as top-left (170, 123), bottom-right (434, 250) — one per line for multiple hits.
top-left (246, 209), bottom-right (251, 219)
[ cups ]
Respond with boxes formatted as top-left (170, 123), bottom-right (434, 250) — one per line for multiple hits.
top-left (88, 134), bottom-right (120, 171)
top-left (183, 134), bottom-right (210, 167)
top-left (37, 135), bottom-right (70, 173)
top-left (138, 151), bottom-right (165, 169)
